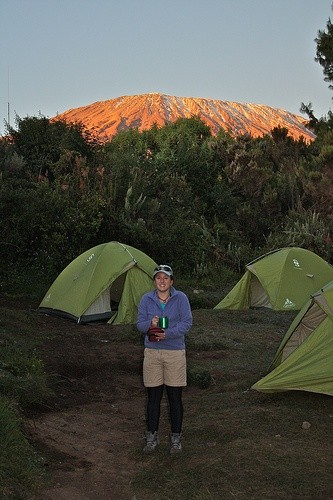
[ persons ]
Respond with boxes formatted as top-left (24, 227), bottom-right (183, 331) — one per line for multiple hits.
top-left (137, 265), bottom-right (193, 453)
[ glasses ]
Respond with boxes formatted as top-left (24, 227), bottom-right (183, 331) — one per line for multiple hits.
top-left (154, 266), bottom-right (172, 273)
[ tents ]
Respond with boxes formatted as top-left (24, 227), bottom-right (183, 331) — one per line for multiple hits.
top-left (37, 240), bottom-right (162, 325)
top-left (242, 280), bottom-right (333, 396)
top-left (213, 245), bottom-right (333, 311)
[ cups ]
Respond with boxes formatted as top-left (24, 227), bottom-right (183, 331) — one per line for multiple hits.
top-left (159, 317), bottom-right (168, 329)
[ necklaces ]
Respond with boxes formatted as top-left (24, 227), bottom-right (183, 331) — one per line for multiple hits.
top-left (158, 294), bottom-right (170, 303)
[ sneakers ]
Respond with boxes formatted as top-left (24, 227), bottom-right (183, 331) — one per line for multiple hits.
top-left (169, 432), bottom-right (184, 455)
top-left (141, 430), bottom-right (160, 454)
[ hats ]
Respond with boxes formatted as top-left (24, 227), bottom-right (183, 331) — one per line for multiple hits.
top-left (152, 264), bottom-right (174, 280)
